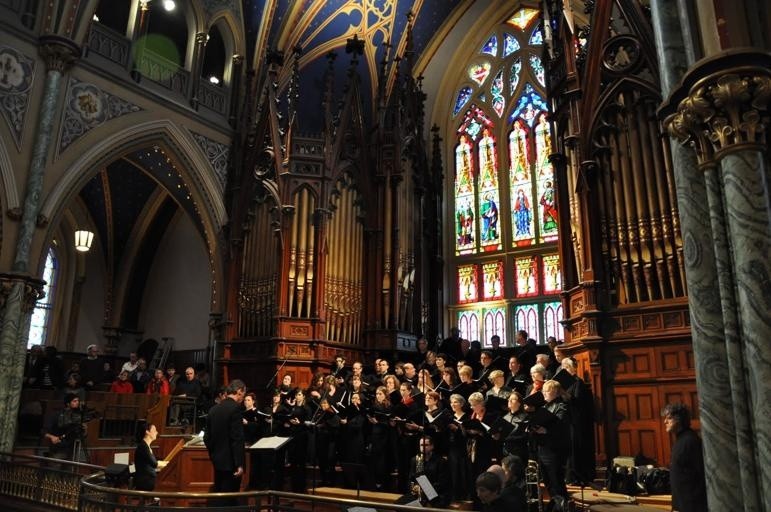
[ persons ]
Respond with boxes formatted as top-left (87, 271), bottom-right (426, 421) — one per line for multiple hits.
top-left (203, 379), bottom-right (249, 505)
top-left (269, 327), bottom-right (599, 510)
top-left (21, 343), bottom-right (211, 427)
top-left (212, 385), bottom-right (230, 407)
top-left (453, 114), bottom-right (558, 246)
top-left (42, 392), bottom-right (89, 482)
top-left (611, 44), bottom-right (632, 68)
top-left (659, 402), bottom-right (711, 512)
top-left (240, 391), bottom-right (263, 445)
top-left (131, 422), bottom-right (170, 512)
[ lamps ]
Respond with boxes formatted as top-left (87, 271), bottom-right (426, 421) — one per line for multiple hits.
top-left (72, 175), bottom-right (95, 253)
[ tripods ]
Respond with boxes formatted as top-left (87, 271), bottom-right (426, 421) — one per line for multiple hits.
top-left (71, 437), bottom-right (96, 483)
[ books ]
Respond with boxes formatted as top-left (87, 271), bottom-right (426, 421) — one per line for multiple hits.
top-left (153, 459), bottom-right (170, 472)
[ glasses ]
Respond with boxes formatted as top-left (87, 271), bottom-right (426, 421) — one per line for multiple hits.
top-left (450, 403), bottom-right (461, 406)
top-left (471, 401), bottom-right (480, 409)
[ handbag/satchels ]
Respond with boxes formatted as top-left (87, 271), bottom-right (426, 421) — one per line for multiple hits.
top-left (606, 464), bottom-right (637, 495)
top-left (640, 468), bottom-right (671, 495)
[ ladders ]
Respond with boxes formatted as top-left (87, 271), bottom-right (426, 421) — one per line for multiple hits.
top-left (139, 337), bottom-right (175, 389)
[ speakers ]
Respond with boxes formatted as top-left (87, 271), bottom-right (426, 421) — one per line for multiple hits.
top-left (105, 464), bottom-right (130, 484)
